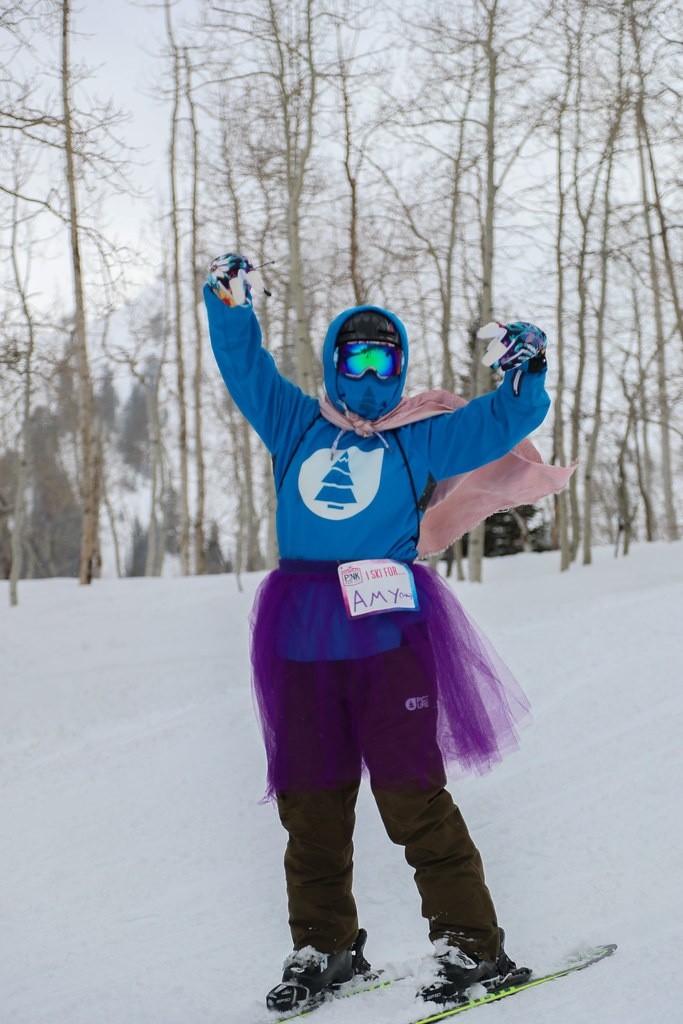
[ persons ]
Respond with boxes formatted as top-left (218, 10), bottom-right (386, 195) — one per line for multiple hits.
top-left (203, 253), bottom-right (552, 1015)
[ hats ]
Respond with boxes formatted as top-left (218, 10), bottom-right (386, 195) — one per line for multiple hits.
top-left (336, 312), bottom-right (401, 345)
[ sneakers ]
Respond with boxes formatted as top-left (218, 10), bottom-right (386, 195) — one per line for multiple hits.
top-left (266, 946), bottom-right (355, 1012)
top-left (416, 952), bottom-right (515, 1002)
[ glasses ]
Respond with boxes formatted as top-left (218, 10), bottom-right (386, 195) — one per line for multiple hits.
top-left (334, 340), bottom-right (405, 380)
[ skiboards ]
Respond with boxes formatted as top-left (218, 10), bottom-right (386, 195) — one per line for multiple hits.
top-left (259, 941), bottom-right (619, 1024)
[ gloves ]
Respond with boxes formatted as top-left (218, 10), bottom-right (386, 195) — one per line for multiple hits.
top-left (209, 253), bottom-right (251, 306)
top-left (476, 324), bottom-right (547, 374)
